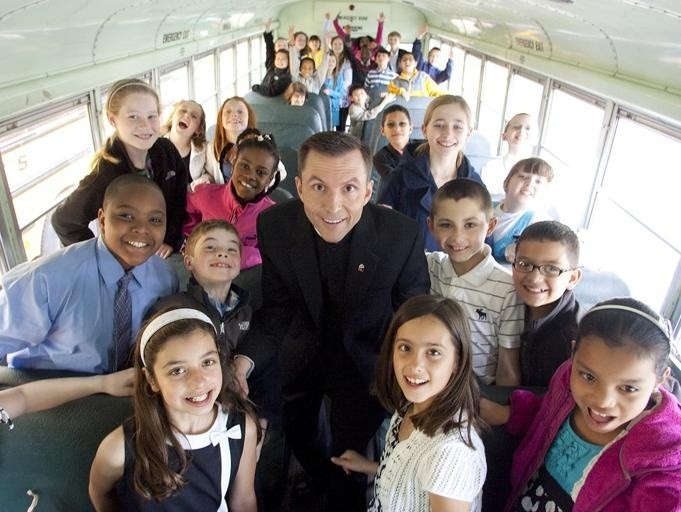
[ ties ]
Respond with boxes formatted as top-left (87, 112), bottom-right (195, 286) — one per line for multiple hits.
top-left (112, 274), bottom-right (134, 371)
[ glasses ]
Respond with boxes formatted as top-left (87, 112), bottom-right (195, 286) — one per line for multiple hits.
top-left (512, 258), bottom-right (578, 276)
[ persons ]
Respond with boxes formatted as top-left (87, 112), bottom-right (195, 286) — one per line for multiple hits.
top-left (480, 112), bottom-right (540, 195)
top-left (512, 221), bottom-right (593, 397)
top-left (388, 52), bottom-right (447, 102)
top-left (385, 31), bottom-right (410, 74)
top-left (485, 157), bottom-right (553, 266)
top-left (480, 297), bottom-right (681, 512)
top-left (159, 100), bottom-right (208, 191)
top-left (185, 128), bottom-right (281, 272)
top-left (413, 31), bottom-right (453, 86)
top-left (347, 83), bottom-right (397, 139)
top-left (291, 32), bottom-right (308, 70)
top-left (344, 40), bottom-right (380, 96)
top-left (322, 51), bottom-right (344, 130)
top-left (330, 293), bottom-right (488, 512)
top-left (287, 25), bottom-right (329, 95)
top-left (275, 36), bottom-right (289, 51)
top-left (334, 11), bottom-right (384, 83)
top-left (365, 49), bottom-right (399, 89)
top-left (284, 82), bottom-right (310, 106)
top-left (373, 104), bottom-right (429, 176)
top-left (330, 36), bottom-right (353, 132)
top-left (257, 130), bottom-right (430, 512)
top-left (142, 219), bottom-right (253, 395)
top-left (375, 95), bottom-right (486, 261)
top-left (304, 35), bottom-right (325, 69)
top-left (0, 175), bottom-right (180, 375)
top-left (251, 18), bottom-right (290, 98)
top-left (51, 77), bottom-right (193, 261)
top-left (424, 178), bottom-right (523, 398)
top-left (0, 367), bottom-right (138, 423)
top-left (192, 97), bottom-right (287, 192)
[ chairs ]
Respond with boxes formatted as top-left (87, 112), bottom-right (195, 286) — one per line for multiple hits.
top-left (247, 102), bottom-right (322, 143)
top-left (1, 396), bottom-right (135, 512)
top-left (207, 120), bottom-right (317, 155)
top-left (318, 91), bottom-right (333, 132)
top-left (234, 261), bottom-right (263, 312)
top-left (573, 270), bottom-right (634, 323)
top-left (363, 109), bottom-right (429, 147)
top-left (247, 92), bottom-right (330, 132)
top-left (467, 156), bottom-right (498, 176)
top-left (477, 384), bottom-right (548, 511)
top-left (277, 144), bottom-right (301, 201)
top-left (348, 94), bottom-right (435, 141)
top-left (372, 127), bottom-right (492, 201)
top-left (266, 186), bottom-right (296, 203)
